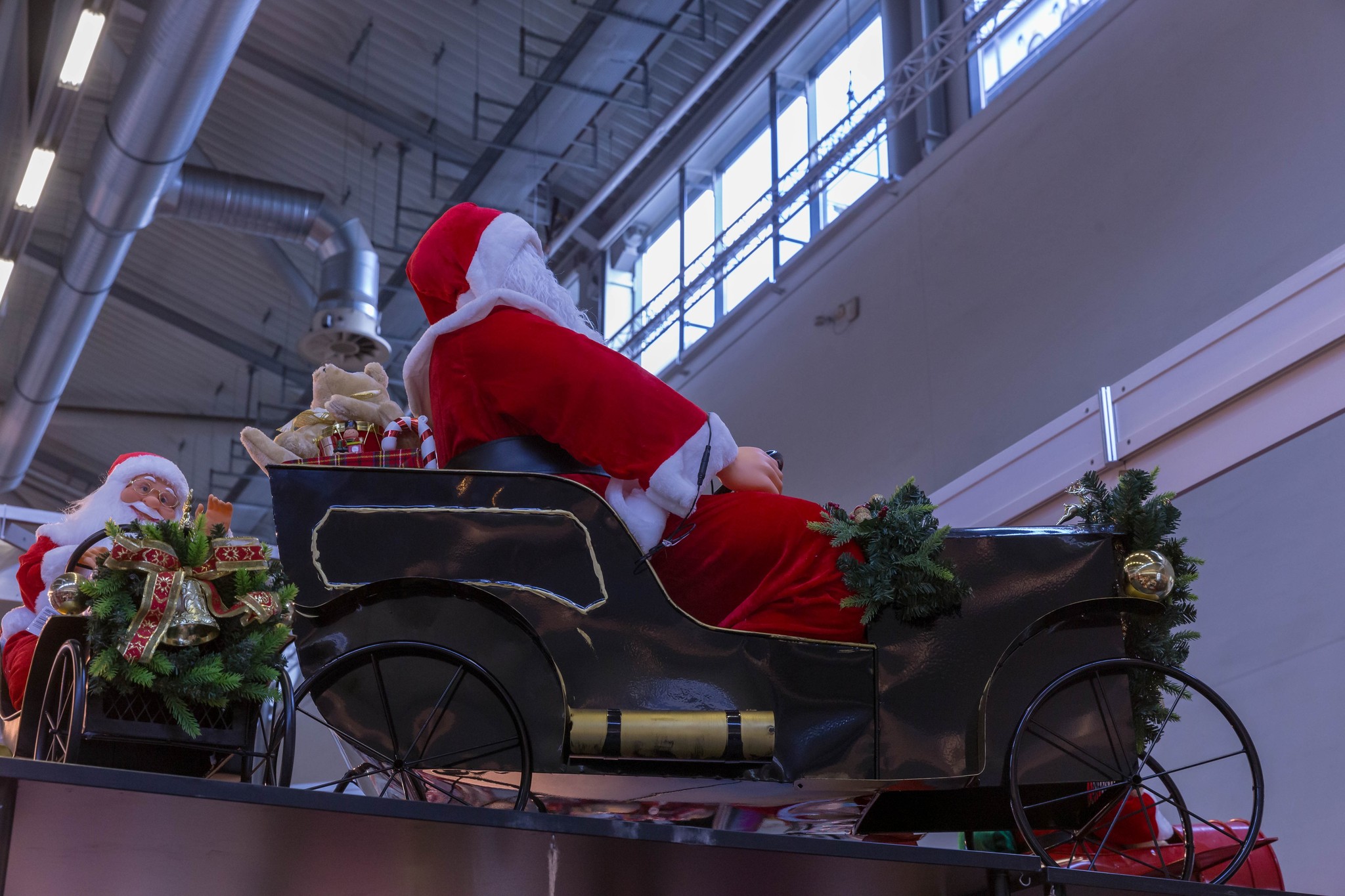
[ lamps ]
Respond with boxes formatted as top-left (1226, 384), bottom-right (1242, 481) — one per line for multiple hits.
top-left (14, 147), bottom-right (55, 213)
top-left (56, 7), bottom-right (106, 91)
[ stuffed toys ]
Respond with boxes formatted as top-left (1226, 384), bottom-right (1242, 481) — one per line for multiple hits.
top-left (399, 202), bottom-right (877, 647)
top-left (240, 361), bottom-right (408, 475)
top-left (1, 451), bottom-right (235, 768)
